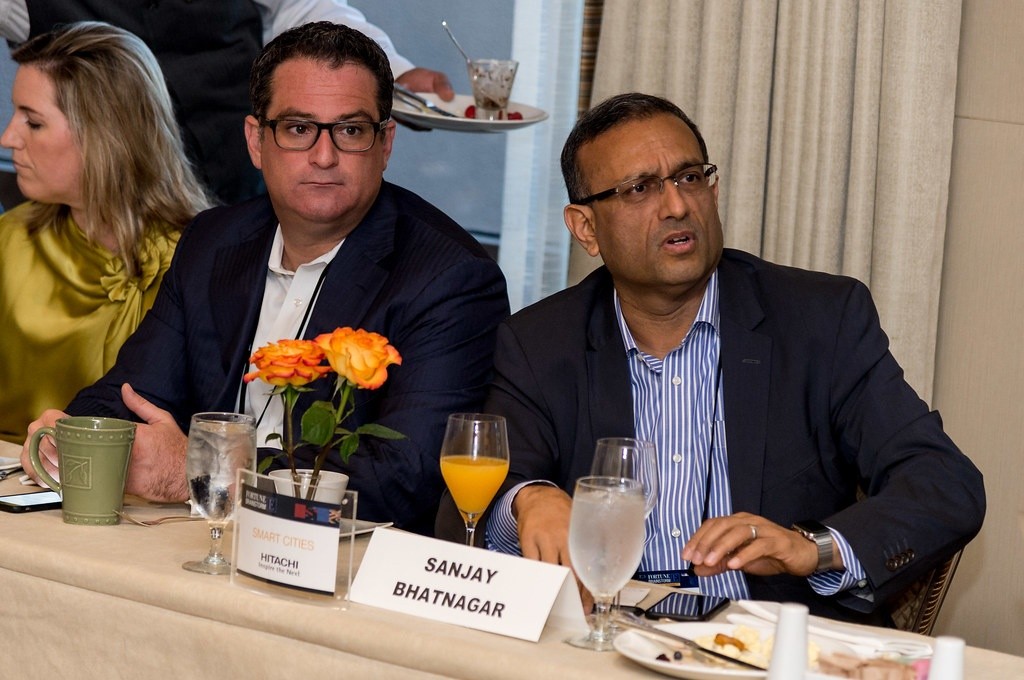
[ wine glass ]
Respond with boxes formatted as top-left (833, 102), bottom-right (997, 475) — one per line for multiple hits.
top-left (586, 437), bottom-right (658, 627)
top-left (439, 414), bottom-right (510, 547)
top-left (182, 412), bottom-right (257, 574)
top-left (563, 476), bottom-right (645, 652)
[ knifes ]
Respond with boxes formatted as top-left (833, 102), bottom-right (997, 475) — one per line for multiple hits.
top-left (394, 83), bottom-right (458, 118)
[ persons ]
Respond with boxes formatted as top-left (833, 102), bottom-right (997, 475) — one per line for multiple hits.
top-left (20, 20), bottom-right (512, 547)
top-left (0, 0), bottom-right (454, 447)
top-left (435, 93), bottom-right (988, 630)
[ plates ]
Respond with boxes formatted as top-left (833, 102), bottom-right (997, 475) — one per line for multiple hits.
top-left (0, 457), bottom-right (22, 470)
top-left (340, 517), bottom-right (393, 538)
top-left (391, 92), bottom-right (548, 131)
top-left (612, 622), bottom-right (828, 680)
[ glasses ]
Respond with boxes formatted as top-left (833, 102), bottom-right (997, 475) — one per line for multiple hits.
top-left (258, 115), bottom-right (391, 152)
top-left (572, 163), bottom-right (718, 206)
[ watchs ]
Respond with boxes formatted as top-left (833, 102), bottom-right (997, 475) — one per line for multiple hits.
top-left (791, 521), bottom-right (834, 575)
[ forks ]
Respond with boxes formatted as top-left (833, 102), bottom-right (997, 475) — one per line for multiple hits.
top-left (113, 510), bottom-right (203, 527)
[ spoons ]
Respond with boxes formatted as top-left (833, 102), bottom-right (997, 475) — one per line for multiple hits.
top-left (442, 21), bottom-right (500, 111)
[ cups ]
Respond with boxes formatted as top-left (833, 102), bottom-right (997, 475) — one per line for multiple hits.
top-left (465, 58), bottom-right (519, 120)
top-left (271, 470), bottom-right (349, 505)
top-left (29, 417), bottom-right (136, 526)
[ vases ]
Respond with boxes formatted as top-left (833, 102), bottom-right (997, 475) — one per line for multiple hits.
top-left (289, 473), bottom-right (321, 500)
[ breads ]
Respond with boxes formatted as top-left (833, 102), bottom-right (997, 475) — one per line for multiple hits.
top-left (815, 652), bottom-right (921, 680)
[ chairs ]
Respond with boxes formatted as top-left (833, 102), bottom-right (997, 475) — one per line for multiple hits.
top-left (892, 545), bottom-right (965, 637)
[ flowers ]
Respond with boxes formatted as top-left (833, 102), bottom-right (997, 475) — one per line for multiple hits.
top-left (244, 327), bottom-right (406, 501)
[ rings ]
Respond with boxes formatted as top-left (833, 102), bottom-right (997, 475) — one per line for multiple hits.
top-left (749, 524), bottom-right (757, 540)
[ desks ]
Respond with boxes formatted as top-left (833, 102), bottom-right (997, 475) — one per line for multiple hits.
top-left (1, 439), bottom-right (1024, 680)
